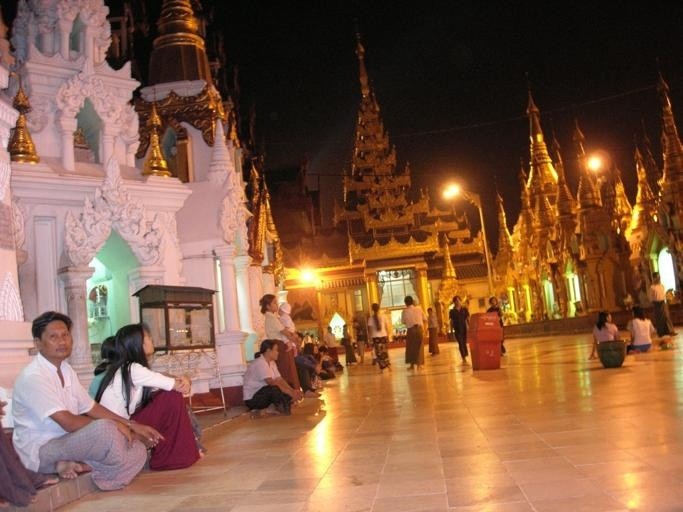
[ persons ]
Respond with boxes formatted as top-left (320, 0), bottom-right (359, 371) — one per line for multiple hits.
top-left (0, 401), bottom-right (60, 504)
top-left (588, 311), bottom-right (622, 360)
top-left (392, 328), bottom-right (408, 345)
top-left (626, 304), bottom-right (655, 351)
top-left (486, 296), bottom-right (507, 356)
top-left (448, 296), bottom-right (470, 361)
top-left (426, 307), bottom-right (440, 356)
top-left (402, 296), bottom-right (424, 371)
top-left (651, 271), bottom-right (678, 338)
top-left (86, 337), bottom-right (200, 438)
top-left (242, 294), bottom-right (376, 417)
top-left (96, 322), bottom-right (202, 469)
top-left (10, 310), bottom-right (165, 490)
top-left (367, 302), bottom-right (394, 373)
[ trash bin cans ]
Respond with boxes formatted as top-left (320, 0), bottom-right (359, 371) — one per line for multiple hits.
top-left (467, 312), bottom-right (503, 370)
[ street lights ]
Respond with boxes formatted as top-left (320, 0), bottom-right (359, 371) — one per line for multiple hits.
top-left (444, 184), bottom-right (493, 295)
